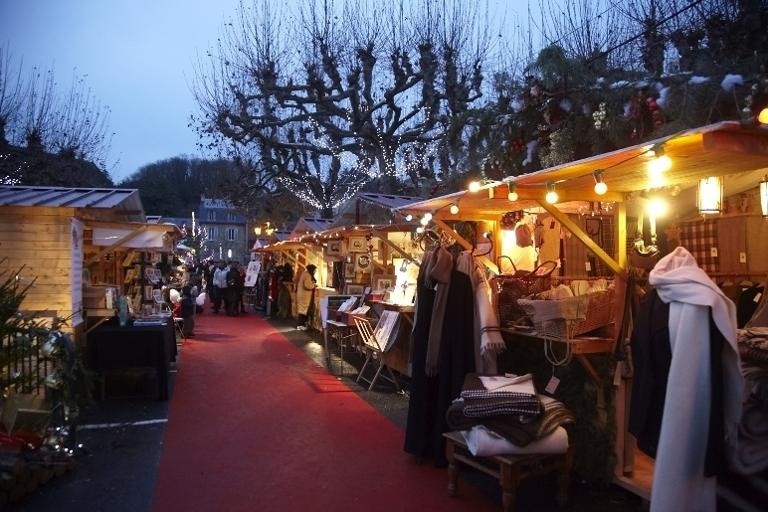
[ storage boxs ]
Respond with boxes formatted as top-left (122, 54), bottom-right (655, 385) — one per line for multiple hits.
top-left (335, 310), bottom-right (346, 321)
top-left (342, 311), bottom-right (366, 325)
top-left (82, 284), bottom-right (121, 308)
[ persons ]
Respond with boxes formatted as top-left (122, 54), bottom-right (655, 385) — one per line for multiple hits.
top-left (204, 260), bottom-right (294, 318)
top-left (297, 264), bottom-right (318, 330)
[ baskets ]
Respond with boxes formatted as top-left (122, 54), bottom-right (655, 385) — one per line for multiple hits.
top-left (497, 279), bottom-right (620, 338)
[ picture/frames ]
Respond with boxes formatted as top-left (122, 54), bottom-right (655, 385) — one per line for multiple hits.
top-left (326, 236), bottom-right (396, 295)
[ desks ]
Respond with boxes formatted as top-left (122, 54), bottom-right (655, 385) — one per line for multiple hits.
top-left (441, 432), bottom-right (577, 512)
top-left (326, 319), bottom-right (379, 379)
top-left (86, 311), bottom-right (177, 400)
top-left (495, 324), bottom-right (616, 422)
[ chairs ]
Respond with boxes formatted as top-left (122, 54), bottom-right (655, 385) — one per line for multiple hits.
top-left (165, 303), bottom-right (185, 339)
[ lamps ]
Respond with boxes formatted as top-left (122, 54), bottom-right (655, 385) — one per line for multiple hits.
top-left (405, 143), bottom-right (672, 232)
top-left (698, 176), bottom-right (721, 214)
top-left (759, 174), bottom-right (768, 220)
